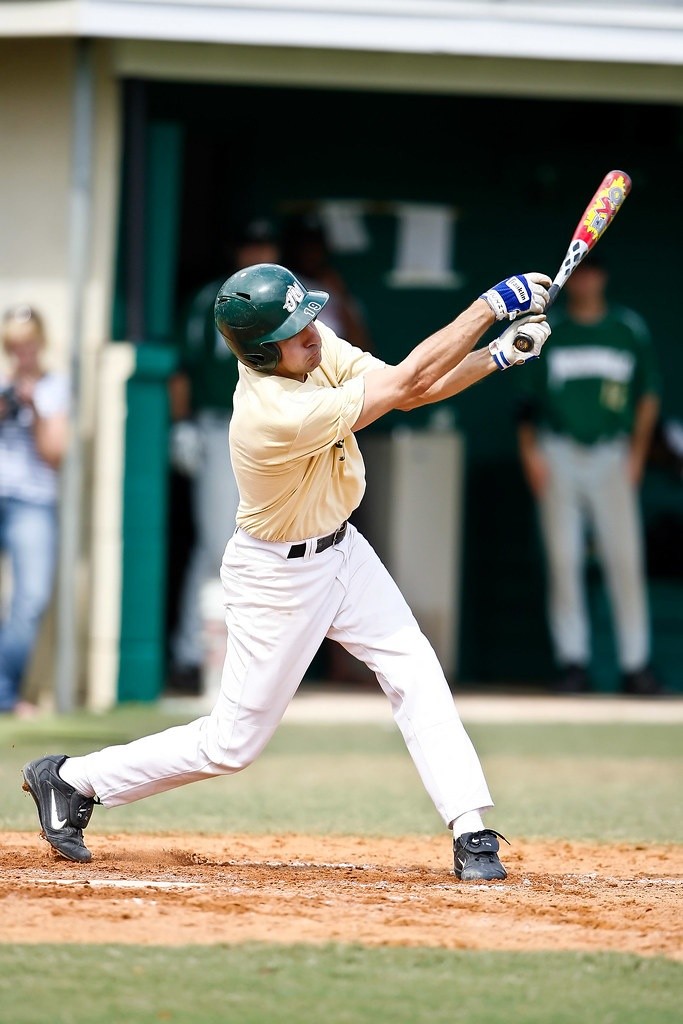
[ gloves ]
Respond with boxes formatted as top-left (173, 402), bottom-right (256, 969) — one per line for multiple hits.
top-left (480, 272), bottom-right (552, 320)
top-left (489, 315), bottom-right (552, 369)
top-left (171, 421), bottom-right (202, 472)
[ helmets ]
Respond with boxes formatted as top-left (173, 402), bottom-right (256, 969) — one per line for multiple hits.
top-left (214, 263), bottom-right (329, 373)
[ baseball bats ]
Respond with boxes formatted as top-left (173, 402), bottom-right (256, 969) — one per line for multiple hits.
top-left (515, 168), bottom-right (632, 353)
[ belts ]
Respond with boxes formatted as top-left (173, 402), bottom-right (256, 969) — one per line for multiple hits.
top-left (287, 520), bottom-right (347, 558)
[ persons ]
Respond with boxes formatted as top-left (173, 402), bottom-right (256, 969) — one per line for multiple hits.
top-left (517, 235), bottom-right (663, 698)
top-left (166, 225), bottom-right (361, 702)
top-left (20, 263), bottom-right (552, 888)
top-left (0, 305), bottom-right (71, 716)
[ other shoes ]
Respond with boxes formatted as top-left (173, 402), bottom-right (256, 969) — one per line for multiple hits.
top-left (615, 670), bottom-right (666, 694)
top-left (552, 668), bottom-right (595, 694)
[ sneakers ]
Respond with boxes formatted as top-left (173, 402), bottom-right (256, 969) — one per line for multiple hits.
top-left (453, 829), bottom-right (511, 881)
top-left (22, 754), bottom-right (102, 862)
